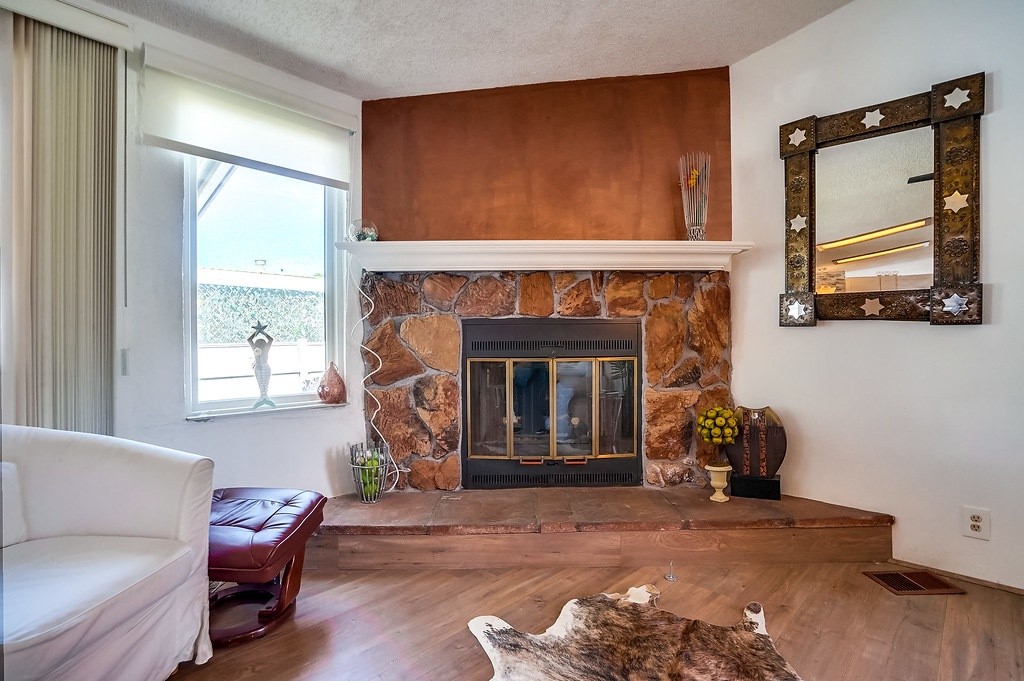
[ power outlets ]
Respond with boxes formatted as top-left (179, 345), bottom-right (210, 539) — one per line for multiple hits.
top-left (962, 505), bottom-right (991, 541)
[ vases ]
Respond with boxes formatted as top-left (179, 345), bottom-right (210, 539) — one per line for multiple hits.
top-left (704, 464), bottom-right (733, 502)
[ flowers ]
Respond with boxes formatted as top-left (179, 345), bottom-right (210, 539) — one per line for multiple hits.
top-left (697, 406), bottom-right (738, 467)
top-left (688, 169), bottom-right (701, 187)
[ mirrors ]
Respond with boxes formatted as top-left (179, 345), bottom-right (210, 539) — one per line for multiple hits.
top-left (779, 70), bottom-right (985, 330)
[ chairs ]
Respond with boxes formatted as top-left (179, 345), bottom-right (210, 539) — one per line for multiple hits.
top-left (0, 423), bottom-right (214, 681)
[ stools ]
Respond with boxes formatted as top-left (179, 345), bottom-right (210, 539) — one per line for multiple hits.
top-left (209, 487), bottom-right (328, 644)
